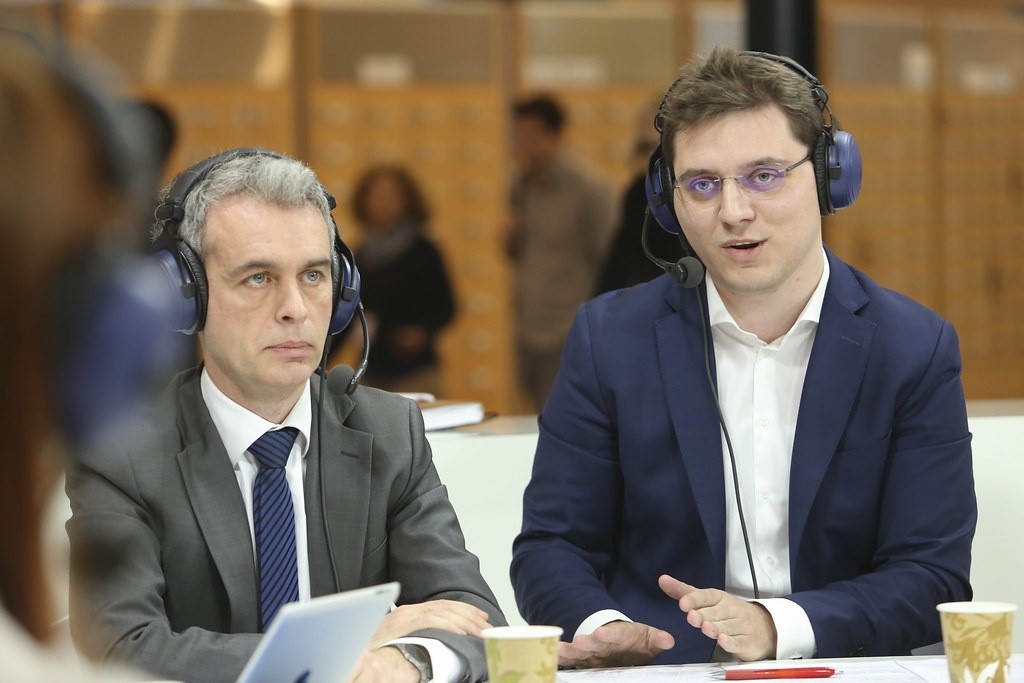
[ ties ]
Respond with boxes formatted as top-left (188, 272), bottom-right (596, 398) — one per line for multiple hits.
top-left (247, 427), bottom-right (301, 635)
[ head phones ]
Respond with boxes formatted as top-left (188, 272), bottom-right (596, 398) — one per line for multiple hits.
top-left (27, 48), bottom-right (199, 454)
top-left (152, 149), bottom-right (361, 335)
top-left (645, 50), bottom-right (863, 235)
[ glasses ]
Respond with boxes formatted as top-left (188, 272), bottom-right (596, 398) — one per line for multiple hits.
top-left (673, 155), bottom-right (813, 204)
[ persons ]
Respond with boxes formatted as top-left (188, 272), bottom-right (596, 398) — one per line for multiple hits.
top-left (65, 147), bottom-right (506, 683)
top-left (332, 160), bottom-right (463, 399)
top-left (508, 44), bottom-right (980, 669)
top-left (489, 92), bottom-right (613, 409)
top-left (0, 6), bottom-right (166, 683)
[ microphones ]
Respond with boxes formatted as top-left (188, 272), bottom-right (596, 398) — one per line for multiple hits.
top-left (327, 311), bottom-right (371, 397)
top-left (642, 217), bottom-right (704, 288)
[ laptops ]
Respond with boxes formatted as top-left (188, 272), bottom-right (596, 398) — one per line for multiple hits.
top-left (234, 583), bottom-right (402, 683)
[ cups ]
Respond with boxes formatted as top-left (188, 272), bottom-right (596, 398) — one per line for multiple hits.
top-left (482, 625), bottom-right (564, 683)
top-left (936, 600), bottom-right (1018, 683)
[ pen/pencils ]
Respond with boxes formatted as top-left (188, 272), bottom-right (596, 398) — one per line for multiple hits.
top-left (706, 666), bottom-right (840, 680)
